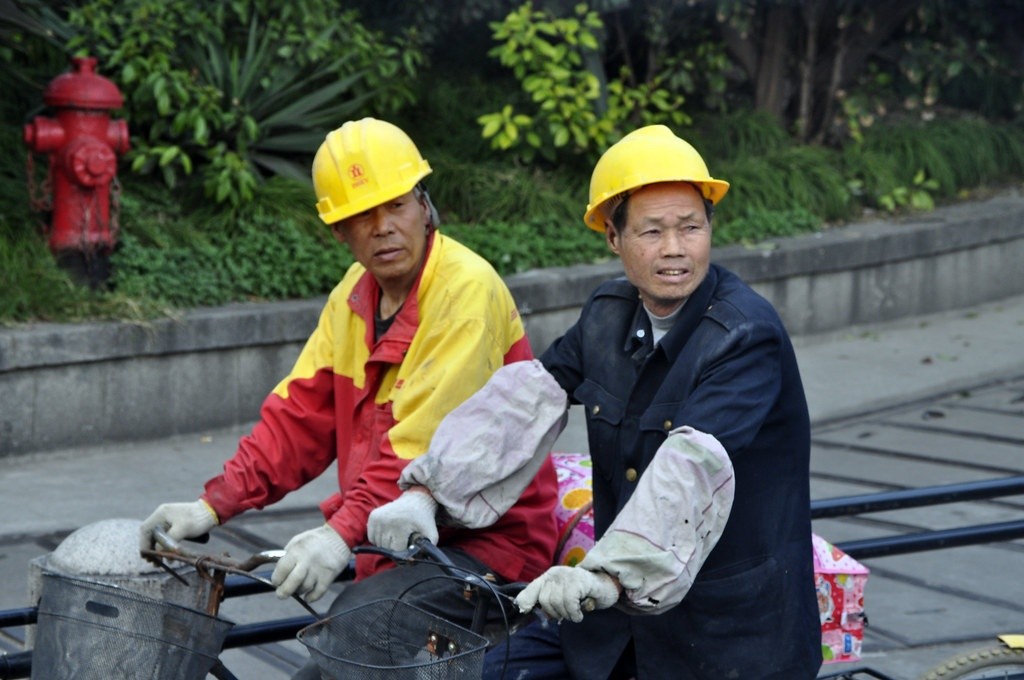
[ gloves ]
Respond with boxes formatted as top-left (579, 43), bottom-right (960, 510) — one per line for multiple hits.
top-left (271, 524), bottom-right (352, 604)
top-left (367, 491), bottom-right (439, 552)
top-left (513, 565), bottom-right (619, 622)
top-left (139, 498), bottom-right (219, 568)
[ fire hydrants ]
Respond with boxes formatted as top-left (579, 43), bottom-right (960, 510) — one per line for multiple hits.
top-left (25, 58), bottom-right (129, 249)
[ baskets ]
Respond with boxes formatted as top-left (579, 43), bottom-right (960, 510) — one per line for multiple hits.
top-left (30, 573), bottom-right (236, 680)
top-left (296, 598), bottom-right (489, 680)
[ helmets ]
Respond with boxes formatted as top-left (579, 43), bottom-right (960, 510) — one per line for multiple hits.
top-left (311, 117), bottom-right (432, 225)
top-left (583, 124), bottom-right (730, 232)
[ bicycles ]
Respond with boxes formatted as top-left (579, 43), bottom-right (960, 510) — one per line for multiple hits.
top-left (30, 530), bottom-right (338, 680)
top-left (295, 532), bottom-right (889, 680)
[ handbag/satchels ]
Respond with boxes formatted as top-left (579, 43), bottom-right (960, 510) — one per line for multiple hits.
top-left (550, 452), bottom-right (870, 665)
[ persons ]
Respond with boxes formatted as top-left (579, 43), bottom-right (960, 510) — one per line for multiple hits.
top-left (367, 125), bottom-right (823, 680)
top-left (139, 117), bottom-right (559, 680)
